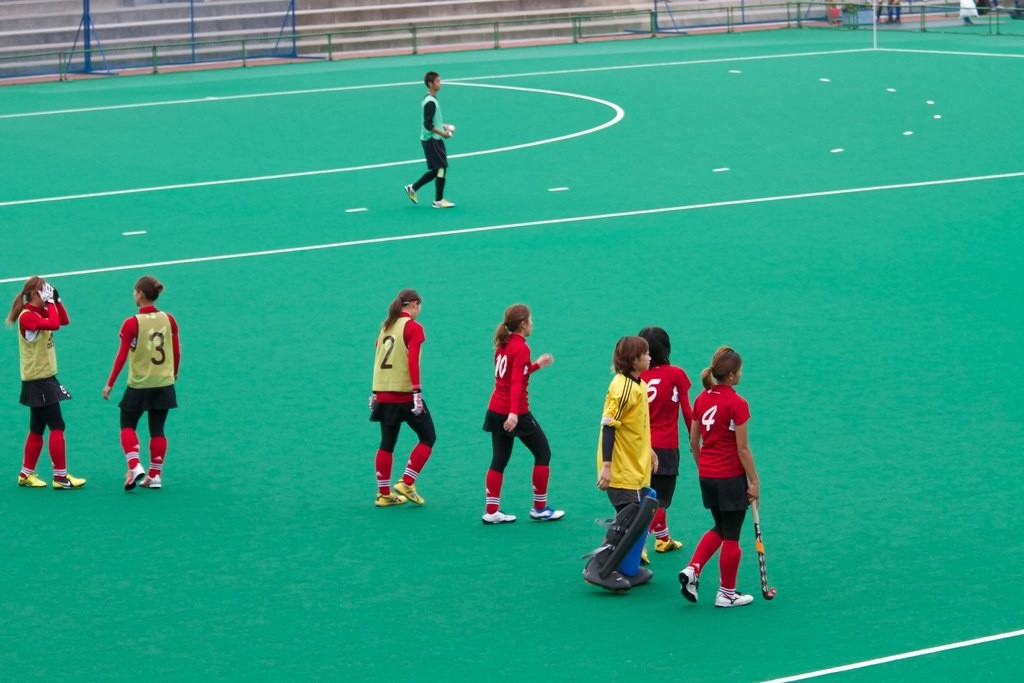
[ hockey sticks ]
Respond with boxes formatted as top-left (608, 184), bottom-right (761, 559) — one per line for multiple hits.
top-left (746, 471), bottom-right (776, 600)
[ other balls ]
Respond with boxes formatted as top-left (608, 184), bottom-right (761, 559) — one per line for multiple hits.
top-left (448, 125), bottom-right (455, 131)
top-left (449, 131), bottom-right (452, 136)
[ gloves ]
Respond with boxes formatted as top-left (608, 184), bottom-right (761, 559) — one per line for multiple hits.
top-left (369, 394), bottom-right (377, 411)
top-left (52, 286), bottom-right (61, 303)
top-left (38, 283), bottom-right (55, 303)
top-left (410, 393), bottom-right (423, 416)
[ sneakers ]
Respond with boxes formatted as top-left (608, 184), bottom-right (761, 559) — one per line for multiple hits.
top-left (530, 506), bottom-right (565, 520)
top-left (124, 463), bottom-right (145, 490)
top-left (641, 547), bottom-right (650, 564)
top-left (404, 184), bottom-right (418, 204)
top-left (482, 509), bottom-right (517, 524)
top-left (18, 474), bottom-right (46, 487)
top-left (52, 474), bottom-right (86, 489)
top-left (655, 538), bottom-right (682, 552)
top-left (393, 479), bottom-right (424, 503)
top-left (715, 589), bottom-right (753, 606)
top-left (139, 475), bottom-right (162, 488)
top-left (375, 492), bottom-right (406, 506)
top-left (432, 198), bottom-right (455, 208)
top-left (679, 566), bottom-right (698, 602)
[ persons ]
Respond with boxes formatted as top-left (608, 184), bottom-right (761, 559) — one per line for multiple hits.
top-left (679, 346), bottom-right (759, 607)
top-left (7, 276), bottom-right (87, 488)
top-left (402, 72), bottom-right (455, 208)
top-left (638, 327), bottom-right (693, 564)
top-left (875, 0), bottom-right (902, 24)
top-left (581, 336), bottom-right (660, 591)
top-left (103, 276), bottom-right (180, 491)
top-left (368, 288), bottom-right (436, 506)
top-left (959, 0), bottom-right (998, 24)
top-left (481, 304), bottom-right (566, 524)
